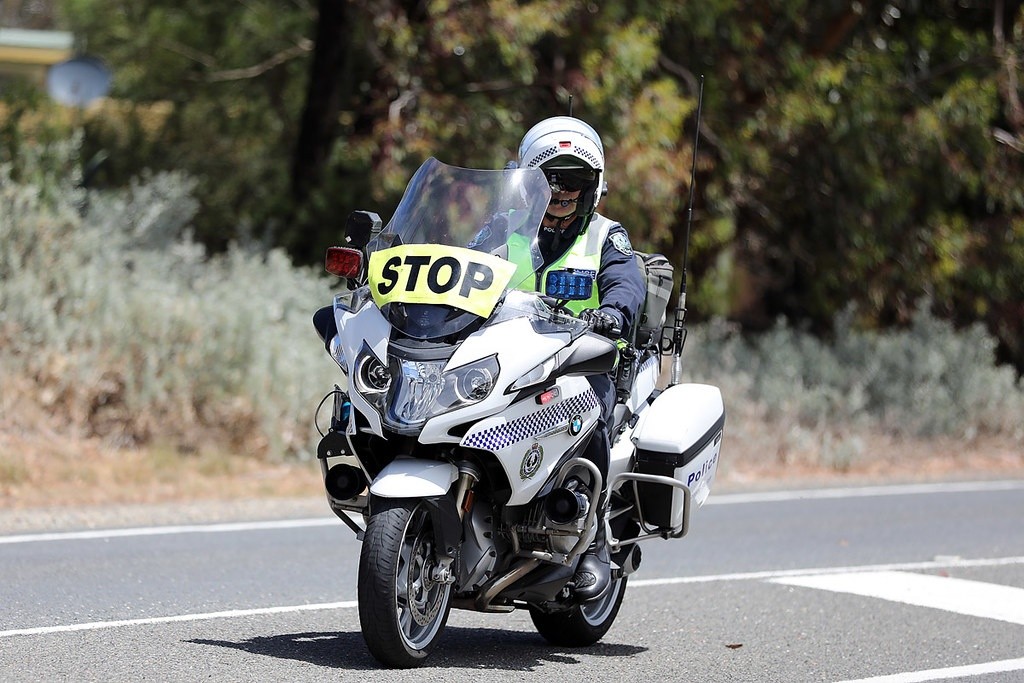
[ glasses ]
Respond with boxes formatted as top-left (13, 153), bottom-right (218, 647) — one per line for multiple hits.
top-left (543, 172), bottom-right (585, 192)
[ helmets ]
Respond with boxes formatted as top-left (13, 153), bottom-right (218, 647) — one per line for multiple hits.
top-left (504, 116), bottom-right (608, 216)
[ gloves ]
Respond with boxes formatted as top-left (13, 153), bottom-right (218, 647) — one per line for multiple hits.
top-left (578, 308), bottom-right (615, 336)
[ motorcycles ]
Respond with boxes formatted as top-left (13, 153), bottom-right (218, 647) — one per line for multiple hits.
top-left (312, 156), bottom-right (724, 671)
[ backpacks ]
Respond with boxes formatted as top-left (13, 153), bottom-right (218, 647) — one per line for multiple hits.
top-left (633, 251), bottom-right (674, 349)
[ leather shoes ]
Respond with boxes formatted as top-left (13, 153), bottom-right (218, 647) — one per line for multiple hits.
top-left (574, 519), bottom-right (611, 603)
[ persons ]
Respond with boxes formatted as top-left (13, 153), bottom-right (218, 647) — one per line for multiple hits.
top-left (470, 116), bottom-right (645, 601)
